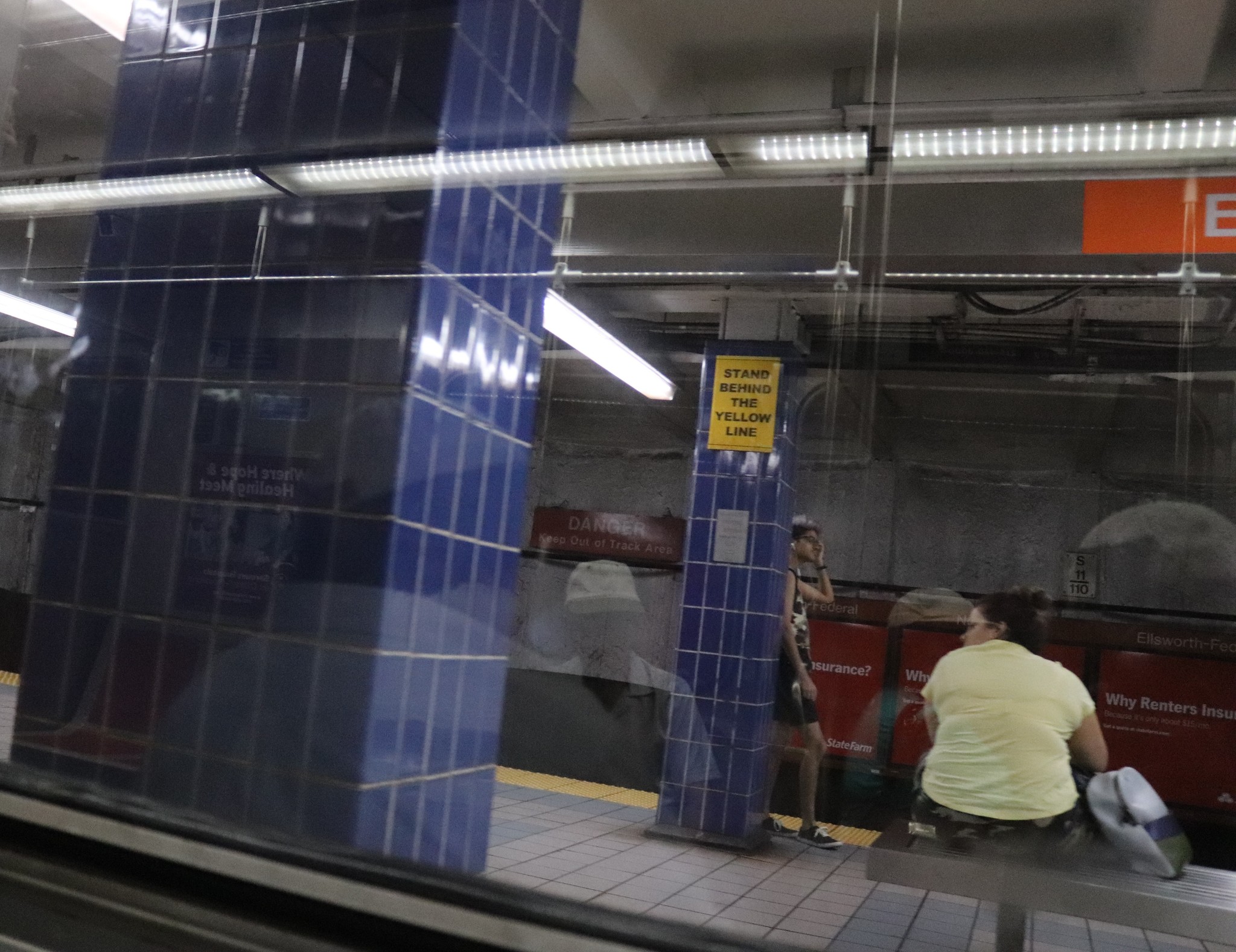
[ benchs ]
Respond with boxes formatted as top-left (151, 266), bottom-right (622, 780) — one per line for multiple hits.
top-left (866, 821), bottom-right (1236, 952)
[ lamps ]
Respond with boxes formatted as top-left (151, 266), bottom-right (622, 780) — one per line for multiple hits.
top-left (0, 276), bottom-right (80, 337)
top-left (543, 283), bottom-right (685, 400)
top-left (0, 92), bottom-right (1236, 221)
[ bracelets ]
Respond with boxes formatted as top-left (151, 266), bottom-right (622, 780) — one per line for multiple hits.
top-left (816, 565), bottom-right (828, 571)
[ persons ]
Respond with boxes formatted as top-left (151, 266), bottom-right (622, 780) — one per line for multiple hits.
top-left (761, 515), bottom-right (844, 847)
top-left (913, 594), bottom-right (1108, 857)
top-left (539, 558), bottom-right (719, 833)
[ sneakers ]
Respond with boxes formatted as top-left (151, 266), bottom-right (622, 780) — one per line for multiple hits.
top-left (765, 815), bottom-right (799, 840)
top-left (797, 825), bottom-right (843, 848)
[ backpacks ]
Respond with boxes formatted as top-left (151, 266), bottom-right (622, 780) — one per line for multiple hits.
top-left (1083, 766), bottom-right (1195, 879)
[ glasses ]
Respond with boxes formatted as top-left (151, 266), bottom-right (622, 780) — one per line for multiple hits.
top-left (798, 535), bottom-right (818, 545)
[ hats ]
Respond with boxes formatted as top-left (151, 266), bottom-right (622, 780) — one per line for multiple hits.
top-left (561, 561), bottom-right (644, 618)
